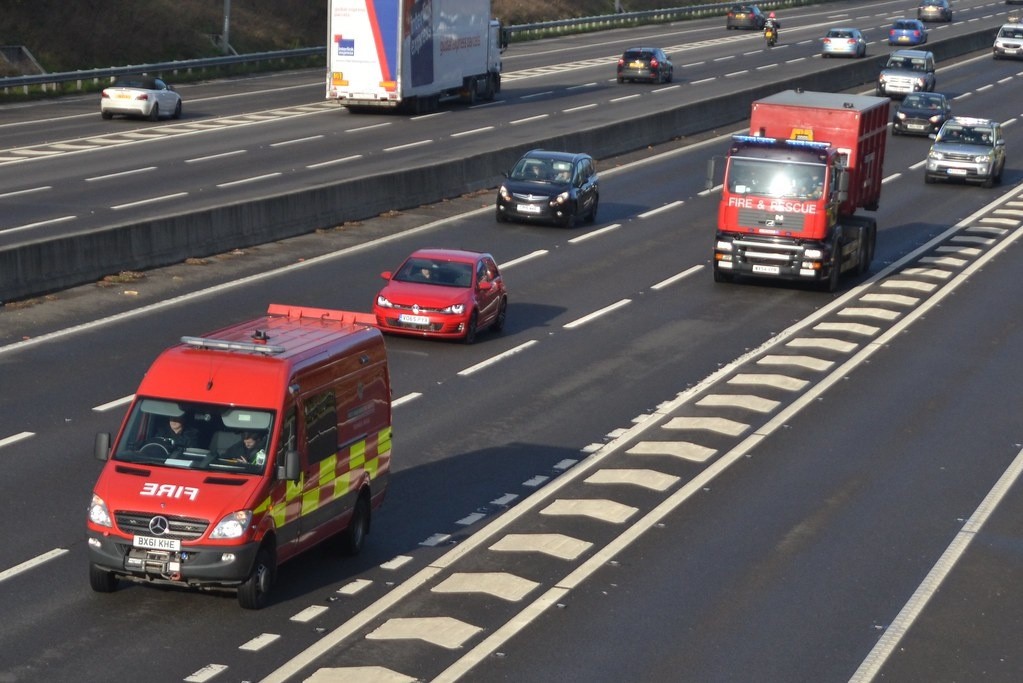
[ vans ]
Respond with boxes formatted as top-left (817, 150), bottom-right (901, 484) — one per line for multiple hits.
top-left (82, 304), bottom-right (395, 609)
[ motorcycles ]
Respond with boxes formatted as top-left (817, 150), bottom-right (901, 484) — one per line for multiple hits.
top-left (764, 20), bottom-right (779, 46)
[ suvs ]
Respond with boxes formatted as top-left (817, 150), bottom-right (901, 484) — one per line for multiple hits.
top-left (876, 49), bottom-right (937, 101)
top-left (924, 116), bottom-right (1005, 186)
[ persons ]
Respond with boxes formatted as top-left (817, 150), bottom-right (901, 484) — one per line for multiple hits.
top-left (416, 267), bottom-right (437, 281)
top-left (524, 164), bottom-right (548, 180)
top-left (945, 130), bottom-right (961, 140)
top-left (155, 416), bottom-right (199, 448)
top-left (763, 12), bottom-right (780, 43)
top-left (908, 100), bottom-right (918, 108)
top-left (555, 166), bottom-right (573, 182)
top-left (1005, 31), bottom-right (1014, 37)
top-left (800, 167), bottom-right (823, 196)
top-left (742, 166), bottom-right (765, 192)
top-left (222, 429), bottom-right (267, 464)
top-left (913, 64), bottom-right (922, 70)
top-left (977, 132), bottom-right (991, 143)
top-left (454, 266), bottom-right (472, 285)
top-left (928, 101), bottom-right (939, 108)
top-left (891, 61), bottom-right (903, 68)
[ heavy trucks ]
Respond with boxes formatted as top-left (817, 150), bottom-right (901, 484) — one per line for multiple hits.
top-left (711, 86), bottom-right (891, 294)
top-left (325, 2), bottom-right (507, 114)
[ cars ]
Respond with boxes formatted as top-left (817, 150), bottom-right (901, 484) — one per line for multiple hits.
top-left (727, 6), bottom-right (765, 31)
top-left (888, 19), bottom-right (928, 46)
top-left (616, 48), bottom-right (674, 84)
top-left (496, 149), bottom-right (599, 229)
top-left (373, 246), bottom-right (508, 345)
top-left (917, 0), bottom-right (953, 22)
top-left (822, 28), bottom-right (865, 58)
top-left (100, 76), bottom-right (182, 122)
top-left (894, 91), bottom-right (951, 138)
top-left (993, 18), bottom-right (1023, 61)
